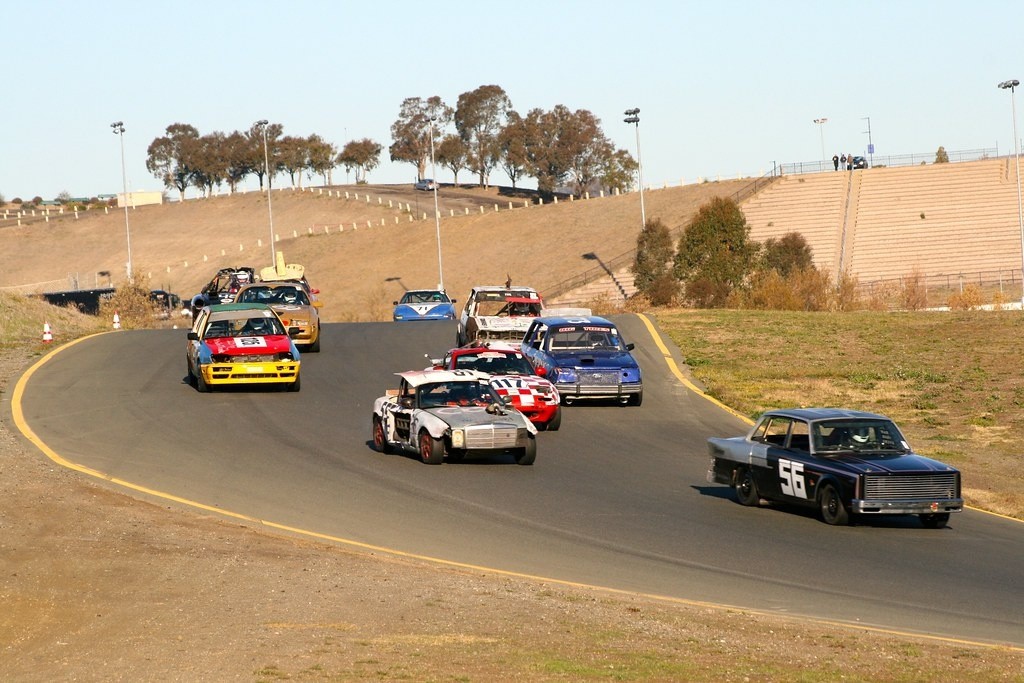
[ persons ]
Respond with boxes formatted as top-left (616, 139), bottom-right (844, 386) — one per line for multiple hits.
top-left (281, 288), bottom-right (298, 305)
top-left (587, 330), bottom-right (607, 345)
top-left (446, 381), bottom-right (489, 406)
top-left (229, 271), bottom-right (250, 293)
top-left (847, 154), bottom-right (854, 170)
top-left (832, 154), bottom-right (839, 170)
top-left (841, 425), bottom-right (870, 446)
top-left (840, 153), bottom-right (847, 170)
top-left (510, 302), bottom-right (535, 317)
top-left (240, 318), bottom-right (271, 336)
top-left (433, 294), bottom-right (442, 301)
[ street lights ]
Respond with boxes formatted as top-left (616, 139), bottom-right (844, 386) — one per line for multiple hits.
top-left (420, 116), bottom-right (443, 289)
top-left (998, 79), bottom-right (1024, 274)
top-left (107, 119), bottom-right (132, 283)
top-left (254, 119), bottom-right (275, 266)
top-left (623, 107), bottom-right (645, 223)
top-left (813, 118), bottom-right (827, 170)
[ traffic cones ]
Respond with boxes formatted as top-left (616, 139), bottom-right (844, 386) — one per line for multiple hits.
top-left (112, 310), bottom-right (121, 330)
top-left (41, 320), bottom-right (53, 348)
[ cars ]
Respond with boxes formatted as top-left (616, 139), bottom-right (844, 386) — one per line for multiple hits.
top-left (847, 156), bottom-right (868, 170)
top-left (393, 289), bottom-right (457, 321)
top-left (456, 285), bottom-right (643, 406)
top-left (191, 252), bottom-right (322, 353)
top-left (186, 303), bottom-right (301, 393)
top-left (372, 339), bottom-right (561, 465)
top-left (707, 407), bottom-right (964, 528)
top-left (416, 178), bottom-right (440, 191)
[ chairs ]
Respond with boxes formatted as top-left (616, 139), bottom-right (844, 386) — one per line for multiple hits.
top-left (205, 321), bottom-right (225, 337)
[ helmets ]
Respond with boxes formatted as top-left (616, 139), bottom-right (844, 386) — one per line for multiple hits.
top-left (249, 318), bottom-right (265, 330)
top-left (470, 384), bottom-right (483, 397)
top-left (589, 332), bottom-right (606, 346)
top-left (847, 427), bottom-right (869, 447)
top-left (433, 293), bottom-right (442, 302)
top-left (518, 304), bottom-right (529, 312)
top-left (237, 271), bottom-right (249, 287)
top-left (284, 286), bottom-right (298, 302)
